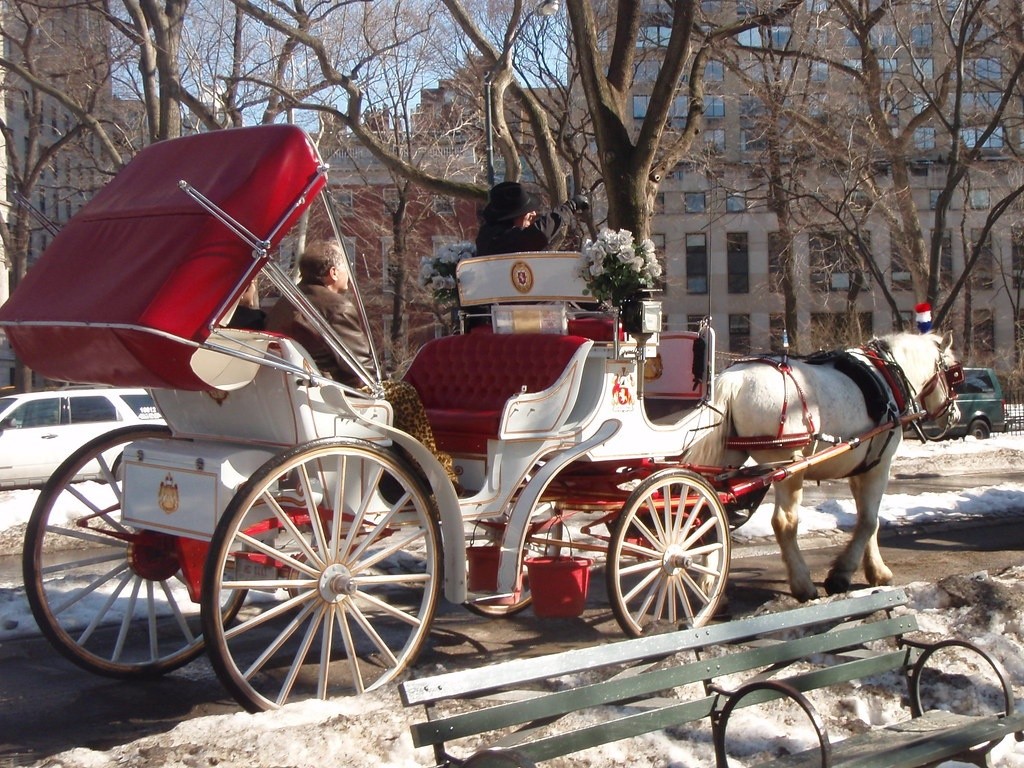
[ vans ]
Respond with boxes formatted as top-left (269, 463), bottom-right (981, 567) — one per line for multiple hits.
top-left (902, 367), bottom-right (1009, 440)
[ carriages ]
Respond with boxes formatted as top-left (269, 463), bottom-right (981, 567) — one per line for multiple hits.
top-left (0, 120), bottom-right (969, 716)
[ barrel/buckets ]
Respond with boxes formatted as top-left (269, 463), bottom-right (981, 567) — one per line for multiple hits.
top-left (523, 515), bottom-right (595, 620)
top-left (466, 513), bottom-right (528, 606)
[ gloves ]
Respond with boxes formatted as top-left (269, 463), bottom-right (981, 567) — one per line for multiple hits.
top-left (570, 195), bottom-right (589, 211)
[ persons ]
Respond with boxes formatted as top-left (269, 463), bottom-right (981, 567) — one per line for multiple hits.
top-left (219, 278), bottom-right (267, 332)
top-left (265, 241), bottom-right (456, 488)
top-left (475, 182), bottom-right (614, 314)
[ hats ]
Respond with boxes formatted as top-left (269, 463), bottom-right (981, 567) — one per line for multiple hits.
top-left (483, 182), bottom-right (543, 221)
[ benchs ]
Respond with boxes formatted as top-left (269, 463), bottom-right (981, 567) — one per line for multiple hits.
top-left (470, 318), bottom-right (627, 348)
top-left (144, 327), bottom-right (393, 452)
top-left (397, 588), bottom-right (1024, 768)
top-left (402, 333), bottom-right (594, 454)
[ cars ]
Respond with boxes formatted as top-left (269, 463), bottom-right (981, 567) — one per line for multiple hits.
top-left (0, 388), bottom-right (170, 489)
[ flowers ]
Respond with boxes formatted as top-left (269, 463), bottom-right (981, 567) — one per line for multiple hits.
top-left (578, 227), bottom-right (662, 307)
top-left (416, 240), bottom-right (477, 309)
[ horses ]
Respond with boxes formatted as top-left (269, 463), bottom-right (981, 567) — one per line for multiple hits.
top-left (680, 326), bottom-right (963, 604)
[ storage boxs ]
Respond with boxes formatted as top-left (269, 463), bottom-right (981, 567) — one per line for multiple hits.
top-left (119, 438), bottom-right (280, 543)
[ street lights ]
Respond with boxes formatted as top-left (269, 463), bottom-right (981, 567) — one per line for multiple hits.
top-left (486, 0), bottom-right (561, 200)
